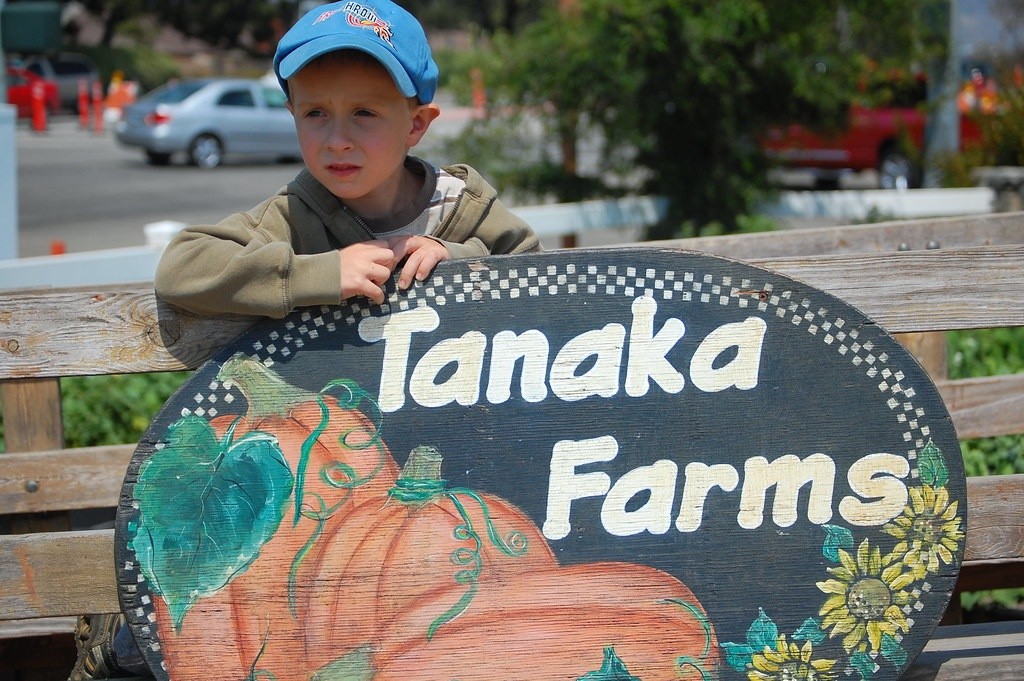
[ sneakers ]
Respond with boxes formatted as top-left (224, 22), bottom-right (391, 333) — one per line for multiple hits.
top-left (67, 613), bottom-right (121, 681)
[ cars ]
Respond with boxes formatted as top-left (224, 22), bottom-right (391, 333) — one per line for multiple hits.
top-left (114, 75), bottom-right (306, 169)
top-left (19, 52), bottom-right (103, 113)
top-left (750, 52), bottom-right (988, 192)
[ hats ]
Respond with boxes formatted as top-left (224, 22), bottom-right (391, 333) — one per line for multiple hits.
top-left (273, 0), bottom-right (439, 104)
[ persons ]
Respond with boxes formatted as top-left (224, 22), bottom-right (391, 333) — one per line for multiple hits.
top-left (61, 0), bottom-right (544, 681)
